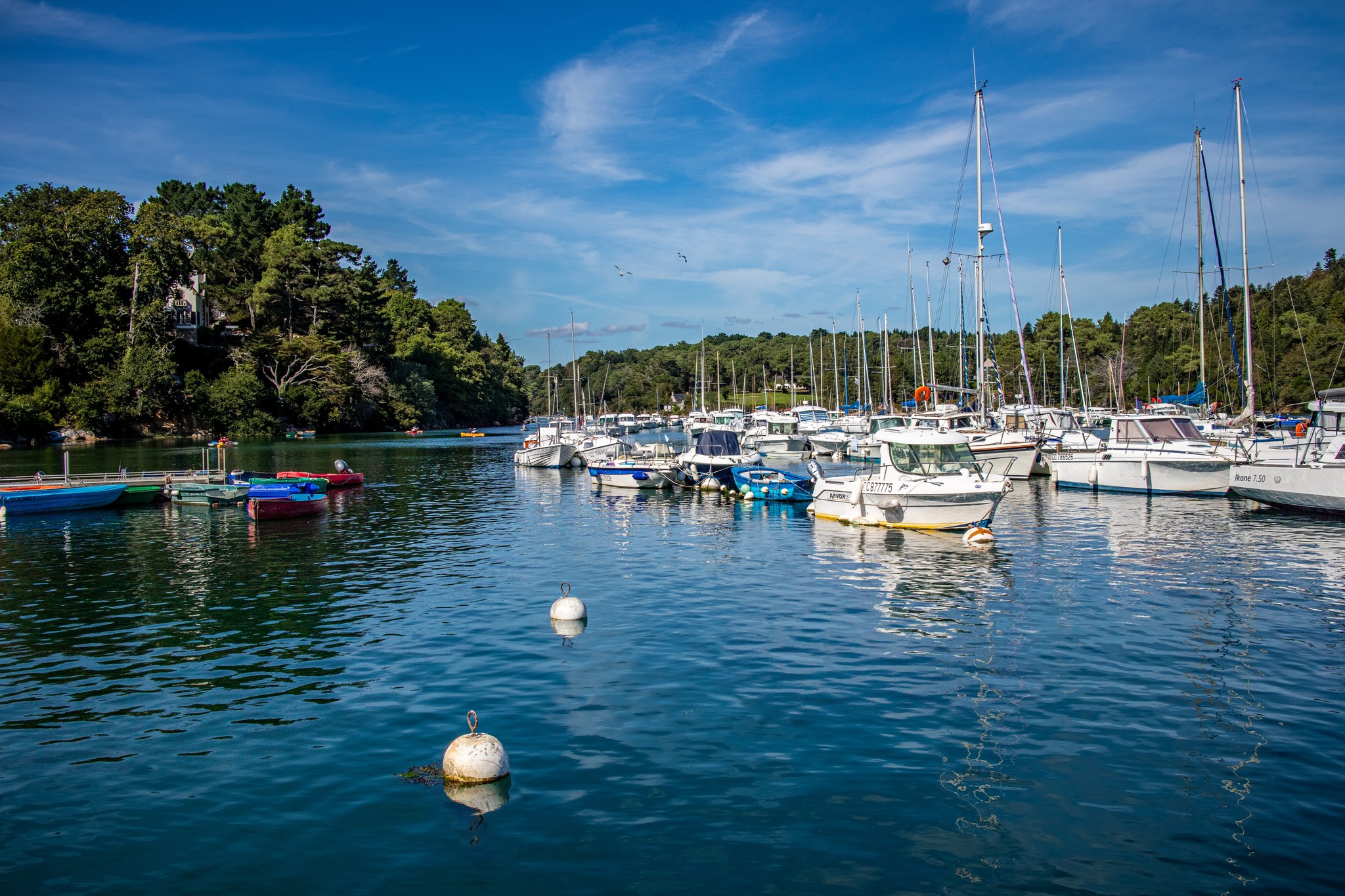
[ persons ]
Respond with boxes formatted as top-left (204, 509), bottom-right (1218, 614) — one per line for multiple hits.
top-left (580, 413), bottom-right (583, 426)
top-left (522, 424), bottom-right (527, 429)
top-left (1205, 400), bottom-right (1224, 418)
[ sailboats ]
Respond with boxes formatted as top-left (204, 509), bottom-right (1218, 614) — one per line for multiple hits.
top-left (513, 46), bottom-right (1345, 532)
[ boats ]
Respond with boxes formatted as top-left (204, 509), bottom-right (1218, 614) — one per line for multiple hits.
top-left (0, 485), bottom-right (130, 514)
top-left (284, 428), bottom-right (316, 437)
top-left (46, 431), bottom-right (67, 443)
top-left (460, 432), bottom-right (485, 437)
top-left (163, 474), bottom-right (250, 506)
top-left (520, 429), bottom-right (530, 431)
top-left (217, 442), bottom-right (230, 448)
top-left (523, 419), bottom-right (535, 423)
top-left (410, 432), bottom-right (417, 435)
top-left (122, 486), bottom-right (162, 503)
top-left (228, 459), bottom-right (364, 521)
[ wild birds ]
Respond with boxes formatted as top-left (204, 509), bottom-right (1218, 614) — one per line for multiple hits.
top-left (677, 252), bottom-right (687, 263)
top-left (614, 265), bottom-right (633, 277)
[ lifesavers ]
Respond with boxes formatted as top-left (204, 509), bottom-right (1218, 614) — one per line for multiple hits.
top-left (1295, 423), bottom-right (1308, 437)
top-left (914, 386), bottom-right (930, 402)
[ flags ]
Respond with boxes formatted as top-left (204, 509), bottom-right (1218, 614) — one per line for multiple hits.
top-left (1137, 400), bottom-right (1142, 409)
top-left (855, 378), bottom-right (859, 385)
top-left (879, 402), bottom-right (883, 407)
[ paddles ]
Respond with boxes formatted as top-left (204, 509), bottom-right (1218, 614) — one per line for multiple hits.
top-left (207, 442), bottom-right (239, 446)
top-left (211, 441), bottom-right (238, 444)
top-left (405, 430), bottom-right (423, 433)
